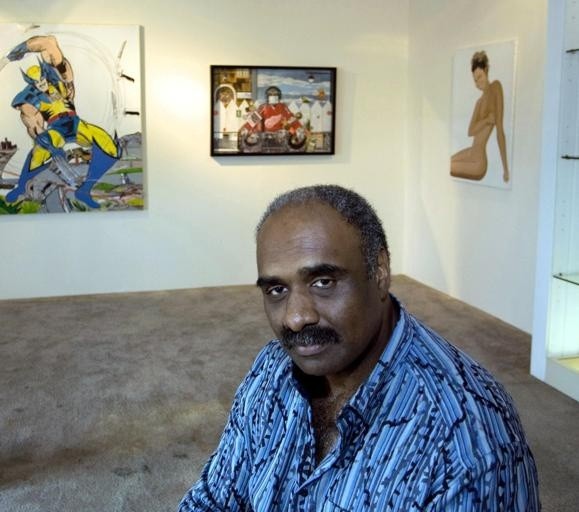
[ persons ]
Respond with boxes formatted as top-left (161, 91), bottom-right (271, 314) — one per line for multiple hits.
top-left (2, 33), bottom-right (121, 211)
top-left (175, 186), bottom-right (543, 510)
top-left (450, 52), bottom-right (511, 182)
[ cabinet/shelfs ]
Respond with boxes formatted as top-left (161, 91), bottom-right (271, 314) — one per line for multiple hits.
top-left (530, 0), bottom-right (579, 402)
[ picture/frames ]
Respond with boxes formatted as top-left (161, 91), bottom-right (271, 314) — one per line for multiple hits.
top-left (210, 65), bottom-right (337, 157)
top-left (446, 36), bottom-right (518, 191)
